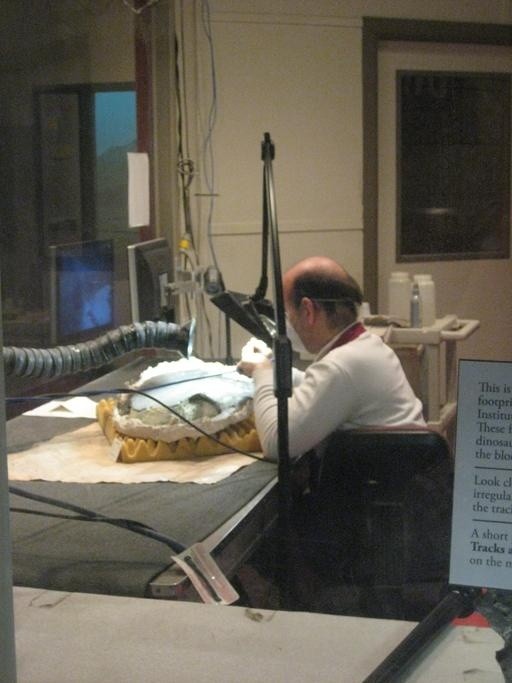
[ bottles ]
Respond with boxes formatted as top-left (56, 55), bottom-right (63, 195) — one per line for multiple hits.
top-left (388, 272), bottom-right (436, 328)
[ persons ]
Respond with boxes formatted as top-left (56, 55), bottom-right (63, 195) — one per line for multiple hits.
top-left (233, 257), bottom-right (430, 618)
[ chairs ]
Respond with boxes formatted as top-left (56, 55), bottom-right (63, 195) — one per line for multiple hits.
top-left (324, 418), bottom-right (459, 614)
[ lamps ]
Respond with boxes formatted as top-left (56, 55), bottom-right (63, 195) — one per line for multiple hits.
top-left (215, 130), bottom-right (336, 605)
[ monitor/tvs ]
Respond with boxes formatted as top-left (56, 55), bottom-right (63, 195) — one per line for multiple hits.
top-left (128, 236), bottom-right (181, 326)
top-left (49, 237), bottom-right (116, 347)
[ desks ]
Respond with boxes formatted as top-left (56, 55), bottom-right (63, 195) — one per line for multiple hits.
top-left (11, 583), bottom-right (505, 683)
top-left (6, 347), bottom-right (337, 600)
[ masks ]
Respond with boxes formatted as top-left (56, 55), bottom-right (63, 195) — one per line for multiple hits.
top-left (285, 320), bottom-right (311, 354)
